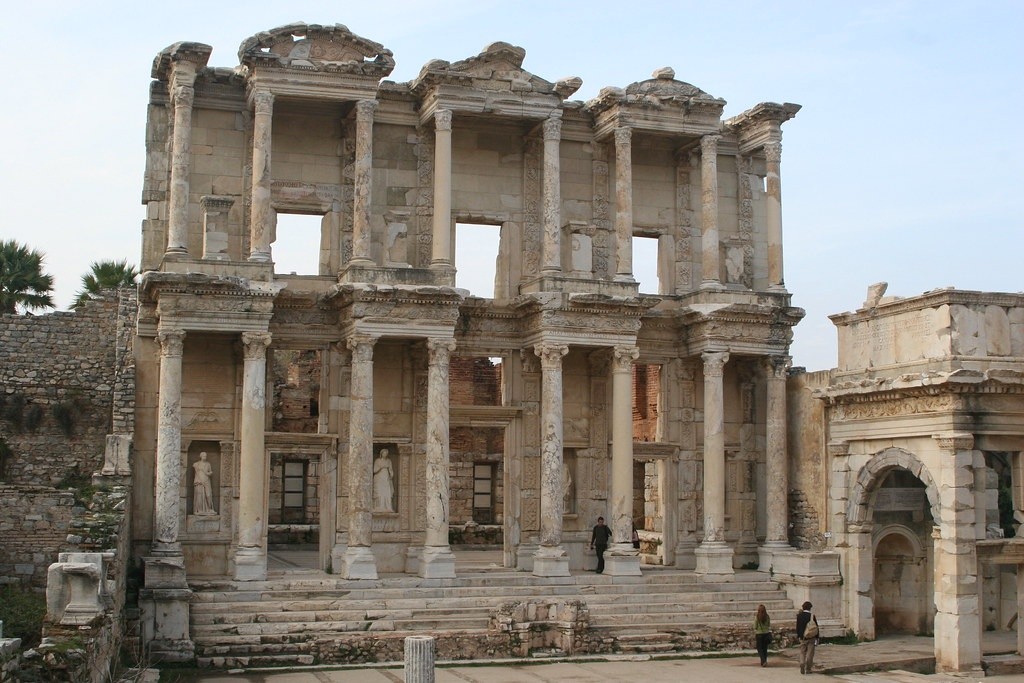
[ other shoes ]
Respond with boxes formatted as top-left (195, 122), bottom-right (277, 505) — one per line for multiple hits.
top-left (806, 669), bottom-right (812, 674)
top-left (800, 664), bottom-right (805, 674)
top-left (761, 662), bottom-right (766, 666)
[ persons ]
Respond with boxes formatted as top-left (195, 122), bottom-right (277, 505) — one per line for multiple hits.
top-left (796, 601), bottom-right (819, 674)
top-left (632, 521), bottom-right (639, 548)
top-left (753, 605), bottom-right (770, 666)
top-left (373, 448), bottom-right (395, 513)
top-left (192, 452), bottom-right (217, 515)
top-left (590, 516), bottom-right (612, 574)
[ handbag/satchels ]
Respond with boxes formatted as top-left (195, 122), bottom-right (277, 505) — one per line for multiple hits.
top-left (768, 632), bottom-right (772, 644)
top-left (632, 530), bottom-right (640, 549)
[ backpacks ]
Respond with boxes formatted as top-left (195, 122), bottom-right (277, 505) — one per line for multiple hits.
top-left (804, 613), bottom-right (818, 639)
